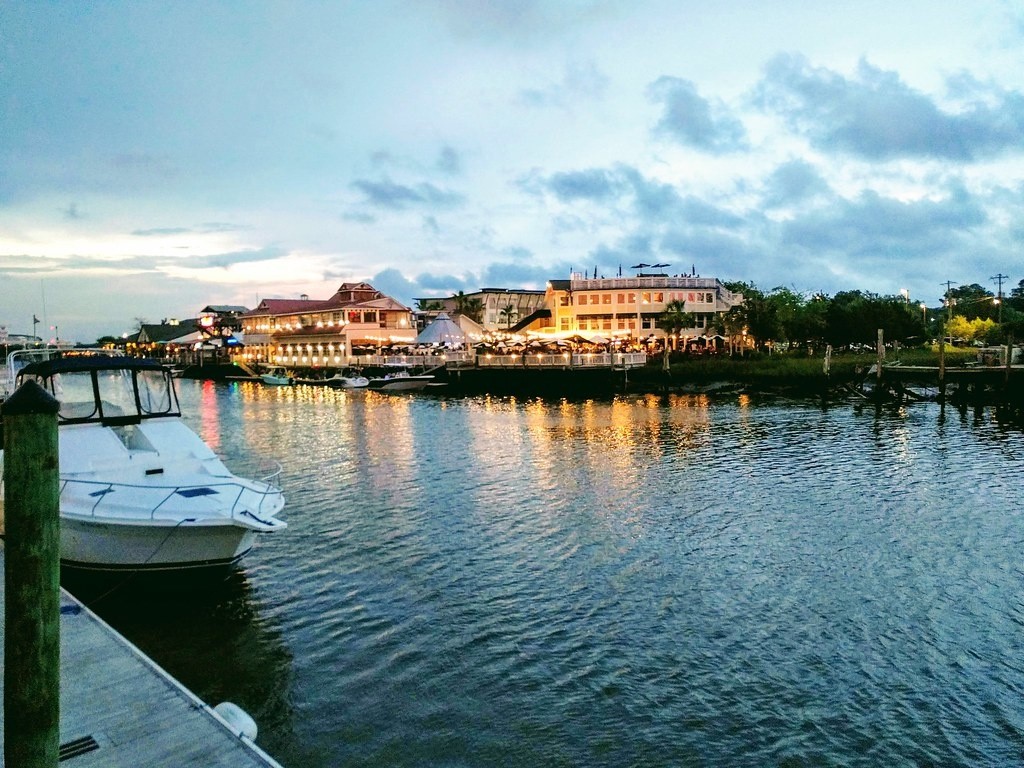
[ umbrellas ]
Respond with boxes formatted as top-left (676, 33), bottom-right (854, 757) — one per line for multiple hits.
top-left (619, 264), bottom-right (621, 277)
top-left (692, 264), bottom-right (695, 275)
top-left (652, 263), bottom-right (672, 274)
top-left (631, 263), bottom-right (651, 273)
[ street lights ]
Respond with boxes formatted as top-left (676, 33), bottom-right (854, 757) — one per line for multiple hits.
top-left (992, 298), bottom-right (1001, 327)
top-left (903, 290), bottom-right (908, 304)
top-left (921, 304), bottom-right (926, 320)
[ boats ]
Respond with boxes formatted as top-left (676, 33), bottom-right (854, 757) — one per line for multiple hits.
top-left (327, 366), bottom-right (369, 389)
top-left (366, 363), bottom-right (435, 390)
top-left (260, 366), bottom-right (296, 385)
top-left (0, 349), bottom-right (289, 572)
